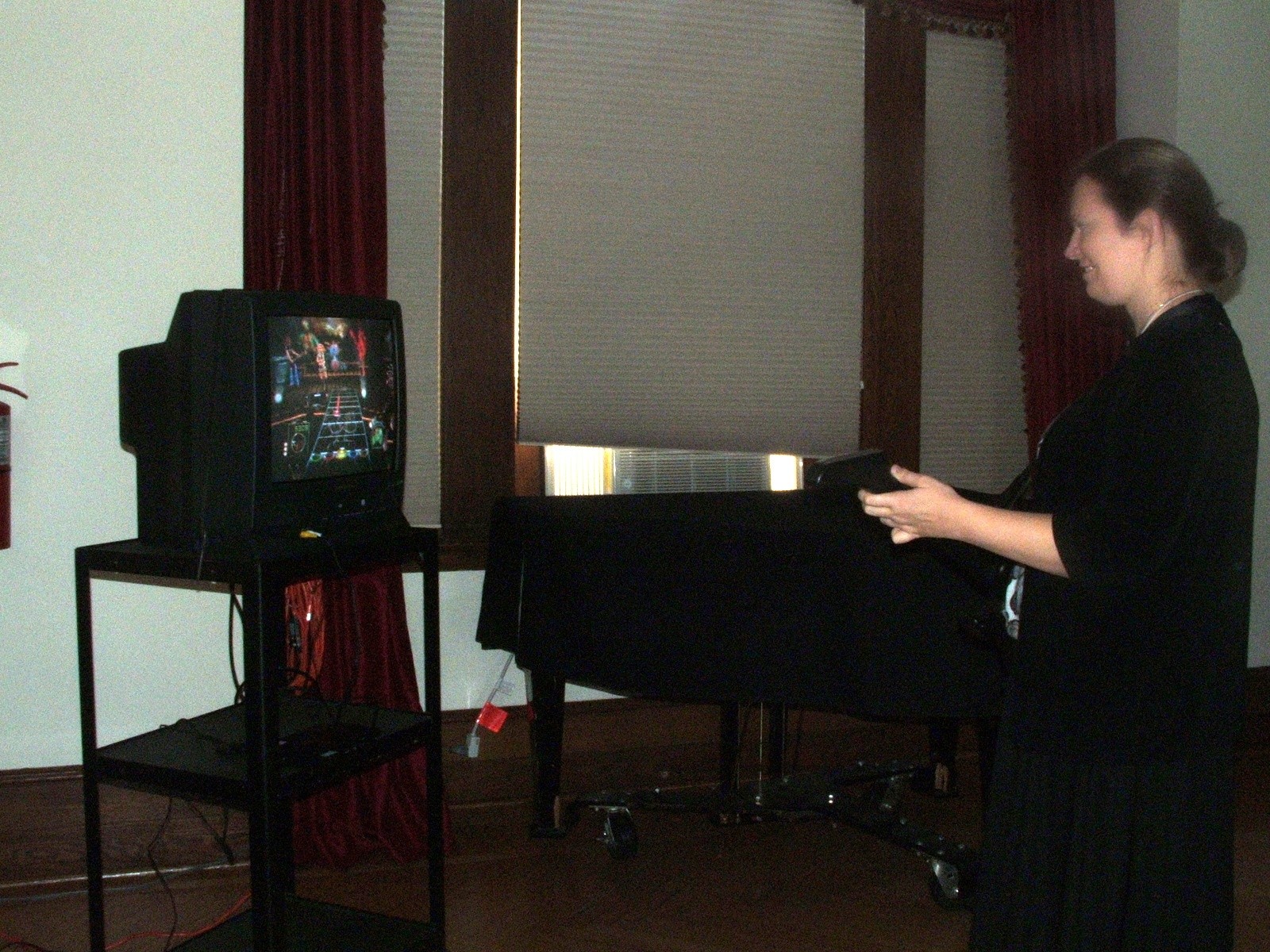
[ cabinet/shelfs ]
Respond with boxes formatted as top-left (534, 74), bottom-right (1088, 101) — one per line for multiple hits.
top-left (76, 525), bottom-right (446, 952)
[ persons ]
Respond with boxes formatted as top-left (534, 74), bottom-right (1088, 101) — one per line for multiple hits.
top-left (857, 137), bottom-right (1261, 952)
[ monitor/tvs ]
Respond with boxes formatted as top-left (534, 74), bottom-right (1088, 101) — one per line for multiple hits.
top-left (119, 287), bottom-right (408, 557)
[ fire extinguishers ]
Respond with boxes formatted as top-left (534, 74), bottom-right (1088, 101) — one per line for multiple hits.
top-left (0, 362), bottom-right (27, 549)
